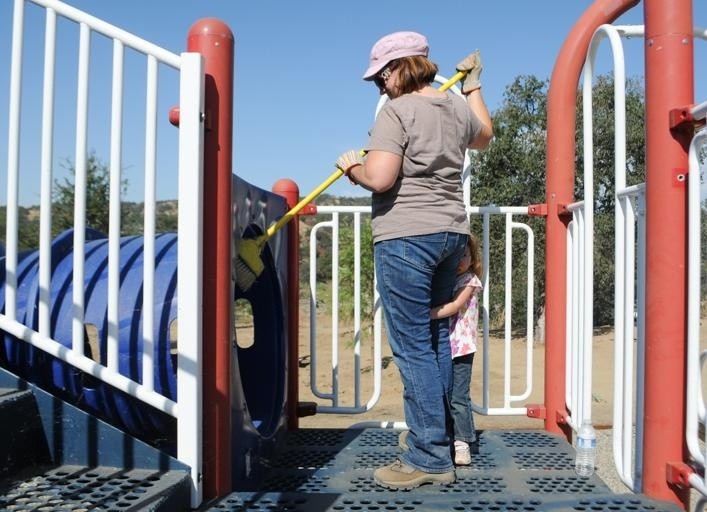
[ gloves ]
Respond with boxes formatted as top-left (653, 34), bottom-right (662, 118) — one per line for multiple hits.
top-left (335, 149), bottom-right (363, 186)
top-left (455, 49), bottom-right (481, 94)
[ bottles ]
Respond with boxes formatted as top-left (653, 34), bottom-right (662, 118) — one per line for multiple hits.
top-left (573, 420), bottom-right (595, 475)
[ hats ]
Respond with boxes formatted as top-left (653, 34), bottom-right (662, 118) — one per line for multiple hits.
top-left (362, 31), bottom-right (428, 80)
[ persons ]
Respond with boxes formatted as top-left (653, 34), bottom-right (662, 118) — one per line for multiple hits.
top-left (335, 31), bottom-right (493, 492)
top-left (429, 233), bottom-right (484, 466)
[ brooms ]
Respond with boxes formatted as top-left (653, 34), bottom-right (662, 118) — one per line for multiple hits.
top-left (230, 69), bottom-right (470, 294)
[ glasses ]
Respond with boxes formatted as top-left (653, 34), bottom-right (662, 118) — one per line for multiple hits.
top-left (373, 61), bottom-right (399, 90)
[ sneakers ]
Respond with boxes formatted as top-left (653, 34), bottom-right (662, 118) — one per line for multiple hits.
top-left (453, 439), bottom-right (471, 465)
top-left (373, 457), bottom-right (455, 490)
top-left (398, 430), bottom-right (409, 451)
